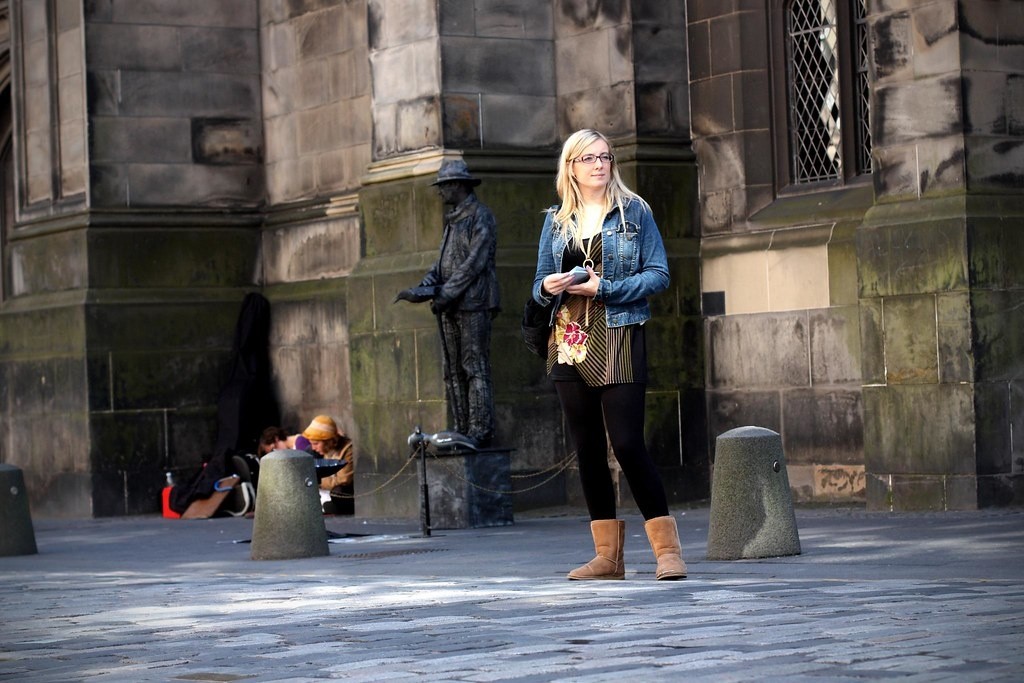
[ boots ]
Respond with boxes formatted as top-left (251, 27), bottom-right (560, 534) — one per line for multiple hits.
top-left (567, 518), bottom-right (626, 579)
top-left (644, 514), bottom-right (687, 580)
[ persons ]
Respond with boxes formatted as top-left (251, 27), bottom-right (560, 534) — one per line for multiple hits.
top-left (258, 428), bottom-right (302, 451)
top-left (392, 161), bottom-right (500, 446)
top-left (531, 129), bottom-right (690, 577)
top-left (302, 415), bottom-right (354, 514)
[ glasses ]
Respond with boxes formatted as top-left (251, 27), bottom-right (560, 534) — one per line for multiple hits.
top-left (565, 152), bottom-right (615, 165)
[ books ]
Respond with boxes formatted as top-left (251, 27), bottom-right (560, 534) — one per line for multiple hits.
top-left (569, 266), bottom-right (599, 284)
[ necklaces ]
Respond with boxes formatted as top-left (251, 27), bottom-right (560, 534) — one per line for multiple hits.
top-left (577, 207), bottom-right (607, 268)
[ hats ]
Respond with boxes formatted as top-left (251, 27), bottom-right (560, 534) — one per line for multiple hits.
top-left (301, 416), bottom-right (337, 440)
